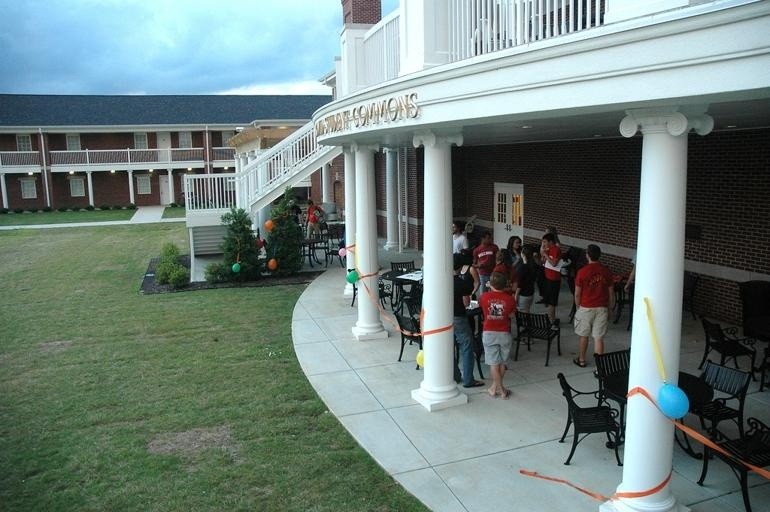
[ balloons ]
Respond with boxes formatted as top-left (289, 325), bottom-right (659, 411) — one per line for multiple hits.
top-left (346, 270), bottom-right (359, 285)
top-left (232, 262), bottom-right (241, 273)
top-left (417, 349), bottom-right (424, 367)
top-left (340, 248), bottom-right (346, 257)
top-left (268, 258), bottom-right (277, 270)
top-left (658, 382), bottom-right (692, 418)
top-left (264, 220), bottom-right (274, 233)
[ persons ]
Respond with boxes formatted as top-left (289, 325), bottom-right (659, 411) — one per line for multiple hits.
top-left (287, 198), bottom-right (303, 223)
top-left (625, 249), bottom-right (636, 331)
top-left (302, 199), bottom-right (325, 237)
top-left (453, 218), bottom-right (613, 400)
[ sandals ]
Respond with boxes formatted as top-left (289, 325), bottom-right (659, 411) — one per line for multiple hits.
top-left (573, 356), bottom-right (587, 367)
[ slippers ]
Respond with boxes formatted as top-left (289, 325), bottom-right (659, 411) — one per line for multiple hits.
top-left (488, 387), bottom-right (511, 400)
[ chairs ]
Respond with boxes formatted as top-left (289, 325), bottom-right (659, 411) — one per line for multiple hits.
top-left (513, 309), bottom-right (562, 367)
top-left (379, 259), bottom-right (423, 318)
top-left (296, 218), bottom-right (346, 268)
top-left (697, 315), bottom-right (759, 382)
top-left (521, 243), bottom-right (541, 254)
top-left (592, 348), bottom-right (631, 439)
top-left (675, 358), bottom-right (769, 512)
top-left (735, 280), bottom-right (769, 392)
top-left (393, 311), bottom-right (424, 370)
top-left (556, 372), bottom-right (624, 467)
top-left (565, 246), bottom-right (634, 332)
top-left (454, 300), bottom-right (485, 380)
top-left (348, 268), bottom-right (358, 307)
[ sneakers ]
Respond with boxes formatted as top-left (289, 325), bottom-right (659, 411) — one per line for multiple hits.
top-left (462, 379), bottom-right (486, 388)
